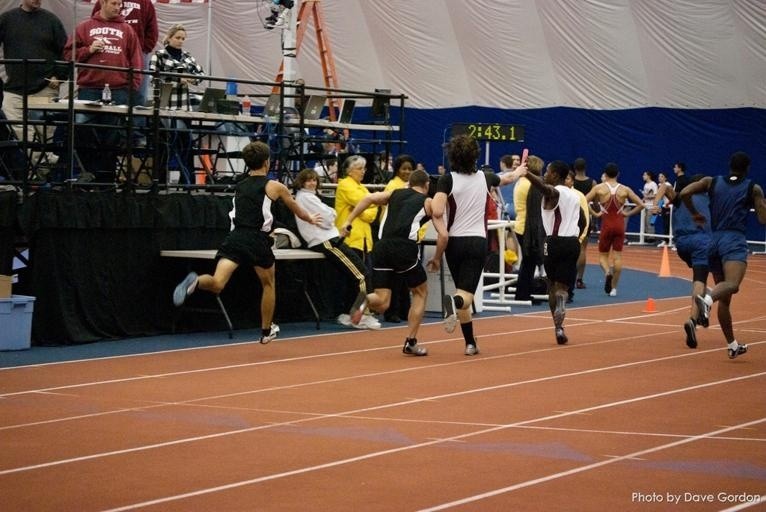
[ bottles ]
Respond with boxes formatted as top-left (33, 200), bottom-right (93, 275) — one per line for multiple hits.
top-left (101, 83), bottom-right (112, 106)
top-left (226, 74), bottom-right (238, 100)
top-left (241, 94), bottom-right (252, 116)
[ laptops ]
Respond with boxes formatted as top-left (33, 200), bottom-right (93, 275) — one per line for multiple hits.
top-left (193, 88), bottom-right (226, 112)
top-left (287, 95), bottom-right (326, 120)
top-left (328, 99), bottom-right (356, 124)
top-left (251, 94), bottom-right (280, 116)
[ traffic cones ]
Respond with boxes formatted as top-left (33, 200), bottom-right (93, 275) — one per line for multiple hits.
top-left (657, 244), bottom-right (671, 278)
top-left (642, 297), bottom-right (658, 314)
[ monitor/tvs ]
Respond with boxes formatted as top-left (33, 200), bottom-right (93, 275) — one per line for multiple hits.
top-left (371, 88), bottom-right (391, 117)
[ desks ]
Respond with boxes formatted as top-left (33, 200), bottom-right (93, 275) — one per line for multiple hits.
top-left (158, 248), bottom-right (326, 341)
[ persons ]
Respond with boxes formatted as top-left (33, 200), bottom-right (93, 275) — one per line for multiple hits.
top-left (526, 160), bottom-right (588, 347)
top-left (63, 0), bottom-right (146, 191)
top-left (289, 154), bottom-right (692, 335)
top-left (430, 134), bottom-right (528, 357)
top-left (679, 152), bottom-right (766, 360)
top-left (0, 0), bottom-right (72, 182)
top-left (152, 24), bottom-right (208, 192)
top-left (651, 172), bottom-right (713, 350)
top-left (342, 169), bottom-right (449, 357)
top-left (172, 140), bottom-right (325, 345)
top-left (91, 0), bottom-right (159, 147)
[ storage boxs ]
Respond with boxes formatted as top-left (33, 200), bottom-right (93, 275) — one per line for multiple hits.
top-left (0, 275), bottom-right (12, 299)
top-left (0, 294), bottom-right (36, 351)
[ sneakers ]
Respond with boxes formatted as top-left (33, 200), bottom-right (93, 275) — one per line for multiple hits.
top-left (694, 294), bottom-right (711, 328)
top-left (402, 336), bottom-right (428, 356)
top-left (553, 327), bottom-right (569, 345)
top-left (441, 294), bottom-right (456, 334)
top-left (348, 292), bottom-right (369, 320)
top-left (26, 153), bottom-right (61, 186)
top-left (172, 271), bottom-right (200, 307)
top-left (337, 312), bottom-right (383, 331)
top-left (604, 272), bottom-right (617, 297)
top-left (71, 172), bottom-right (96, 190)
top-left (684, 318), bottom-right (698, 349)
top-left (553, 289), bottom-right (566, 331)
top-left (577, 278), bottom-right (587, 288)
top-left (258, 324), bottom-right (281, 345)
top-left (464, 342), bottom-right (481, 356)
top-left (644, 238), bottom-right (677, 250)
top-left (727, 342), bottom-right (749, 360)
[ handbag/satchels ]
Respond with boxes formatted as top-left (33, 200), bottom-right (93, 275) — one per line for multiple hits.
top-left (203, 172), bottom-right (250, 193)
